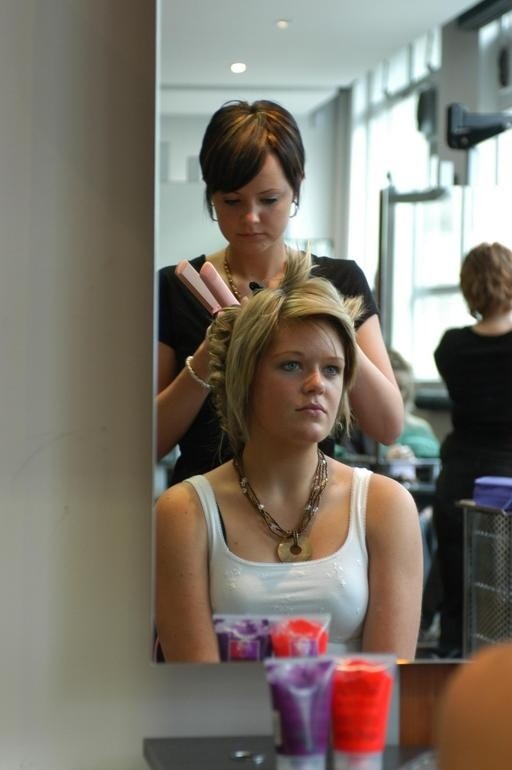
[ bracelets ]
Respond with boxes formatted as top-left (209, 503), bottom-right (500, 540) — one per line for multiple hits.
top-left (185, 355), bottom-right (215, 390)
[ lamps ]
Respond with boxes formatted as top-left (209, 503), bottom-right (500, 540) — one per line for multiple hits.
top-left (446, 100), bottom-right (512, 150)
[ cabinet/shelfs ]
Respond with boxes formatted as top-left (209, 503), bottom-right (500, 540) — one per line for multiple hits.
top-left (457, 499), bottom-right (512, 659)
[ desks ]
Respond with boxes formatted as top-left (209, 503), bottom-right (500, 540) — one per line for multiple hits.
top-left (144, 735), bottom-right (437, 770)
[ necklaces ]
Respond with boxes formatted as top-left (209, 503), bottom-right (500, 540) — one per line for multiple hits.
top-left (232, 444), bottom-right (329, 563)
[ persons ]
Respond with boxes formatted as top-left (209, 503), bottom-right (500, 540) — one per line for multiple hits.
top-left (155, 98), bottom-right (405, 483)
top-left (343, 348), bottom-right (440, 461)
top-left (155, 248), bottom-right (426, 662)
top-left (433, 242), bottom-right (511, 657)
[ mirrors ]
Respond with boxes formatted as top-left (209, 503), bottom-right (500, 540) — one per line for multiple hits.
top-left (153, 0), bottom-right (512, 663)
